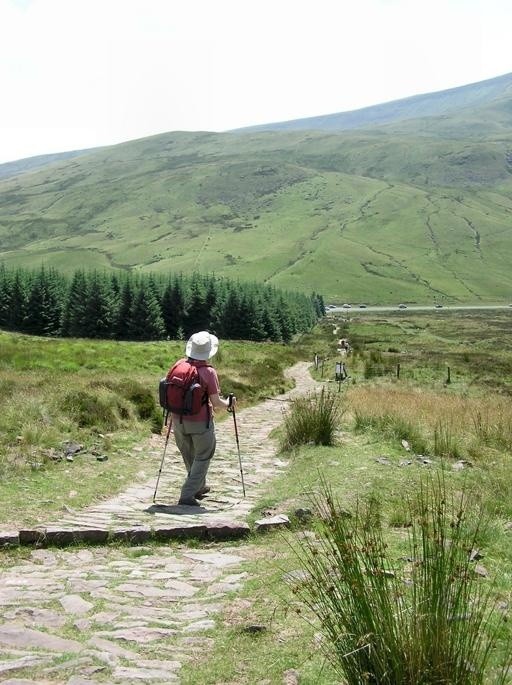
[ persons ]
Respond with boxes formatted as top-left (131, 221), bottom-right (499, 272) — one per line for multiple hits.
top-left (159, 330), bottom-right (236, 506)
top-left (339, 341), bottom-right (349, 350)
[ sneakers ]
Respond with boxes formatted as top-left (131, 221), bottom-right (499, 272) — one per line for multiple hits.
top-left (177, 495), bottom-right (202, 508)
top-left (196, 487), bottom-right (212, 499)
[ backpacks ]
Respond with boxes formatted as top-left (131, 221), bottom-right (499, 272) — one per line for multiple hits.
top-left (157, 358), bottom-right (203, 417)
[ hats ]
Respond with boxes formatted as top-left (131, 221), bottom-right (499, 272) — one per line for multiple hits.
top-left (183, 331), bottom-right (220, 361)
